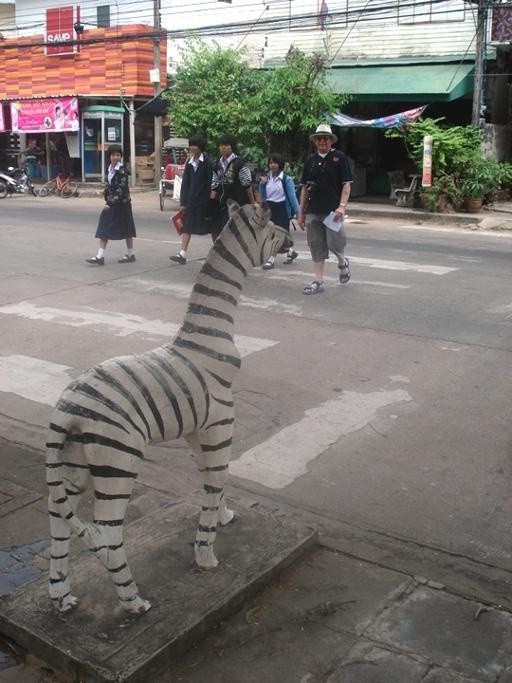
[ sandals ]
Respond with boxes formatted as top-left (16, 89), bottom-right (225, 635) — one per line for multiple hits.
top-left (303, 281), bottom-right (324, 295)
top-left (338, 258), bottom-right (351, 284)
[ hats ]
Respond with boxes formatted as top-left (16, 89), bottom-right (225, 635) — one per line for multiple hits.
top-left (309, 124), bottom-right (337, 145)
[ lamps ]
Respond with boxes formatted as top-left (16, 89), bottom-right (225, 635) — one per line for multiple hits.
top-left (74, 22), bottom-right (104, 34)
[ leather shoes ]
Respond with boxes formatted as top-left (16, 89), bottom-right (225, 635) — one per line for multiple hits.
top-left (170, 254), bottom-right (186, 265)
top-left (85, 257), bottom-right (104, 265)
top-left (283, 251), bottom-right (297, 264)
top-left (118, 254), bottom-right (135, 262)
top-left (263, 261), bottom-right (274, 270)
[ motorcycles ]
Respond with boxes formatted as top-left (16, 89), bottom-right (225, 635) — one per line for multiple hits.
top-left (0, 159), bottom-right (38, 200)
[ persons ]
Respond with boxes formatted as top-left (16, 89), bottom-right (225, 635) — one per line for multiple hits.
top-left (295, 119), bottom-right (354, 299)
top-left (167, 133), bottom-right (216, 266)
top-left (208, 130), bottom-right (257, 243)
top-left (54, 104), bottom-right (68, 128)
top-left (85, 141), bottom-right (140, 267)
top-left (67, 110), bottom-right (79, 128)
top-left (258, 148), bottom-right (301, 271)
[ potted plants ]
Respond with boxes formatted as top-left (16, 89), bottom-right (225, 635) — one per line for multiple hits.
top-left (384, 117), bottom-right (512, 213)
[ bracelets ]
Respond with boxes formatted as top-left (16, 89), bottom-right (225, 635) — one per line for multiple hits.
top-left (339, 201), bottom-right (347, 208)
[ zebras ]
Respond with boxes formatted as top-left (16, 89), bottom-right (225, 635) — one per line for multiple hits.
top-left (44, 196), bottom-right (295, 615)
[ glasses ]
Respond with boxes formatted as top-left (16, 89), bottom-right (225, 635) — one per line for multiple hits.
top-left (315, 136), bottom-right (330, 141)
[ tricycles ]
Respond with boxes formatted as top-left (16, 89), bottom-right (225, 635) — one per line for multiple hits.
top-left (156, 135), bottom-right (192, 212)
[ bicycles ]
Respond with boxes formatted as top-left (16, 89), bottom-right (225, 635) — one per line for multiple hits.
top-left (39, 168), bottom-right (79, 198)
